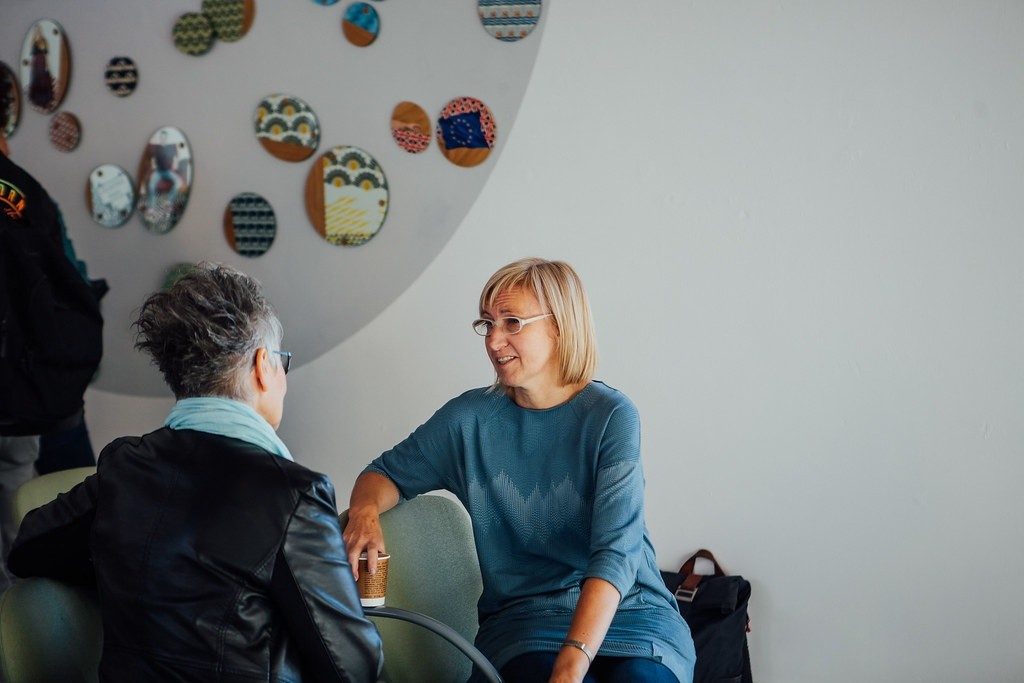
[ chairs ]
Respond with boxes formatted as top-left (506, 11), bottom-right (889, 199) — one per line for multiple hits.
top-left (0, 465), bottom-right (508, 683)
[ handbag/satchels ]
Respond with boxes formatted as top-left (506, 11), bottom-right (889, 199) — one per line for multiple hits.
top-left (659, 549), bottom-right (753, 683)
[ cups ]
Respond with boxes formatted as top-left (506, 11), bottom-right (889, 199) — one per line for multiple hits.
top-left (355, 552), bottom-right (391, 607)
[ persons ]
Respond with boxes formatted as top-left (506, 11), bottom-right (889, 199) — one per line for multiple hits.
top-left (6, 261), bottom-right (387, 682)
top-left (0, 128), bottom-right (104, 501)
top-left (340, 258), bottom-right (697, 683)
top-left (28, 24), bottom-right (54, 108)
top-left (143, 131), bottom-right (187, 234)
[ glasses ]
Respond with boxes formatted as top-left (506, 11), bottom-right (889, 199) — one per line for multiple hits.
top-left (252, 350), bottom-right (291, 375)
top-left (472, 314), bottom-right (554, 336)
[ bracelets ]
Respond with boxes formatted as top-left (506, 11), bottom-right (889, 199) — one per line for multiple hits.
top-left (560, 640), bottom-right (595, 664)
